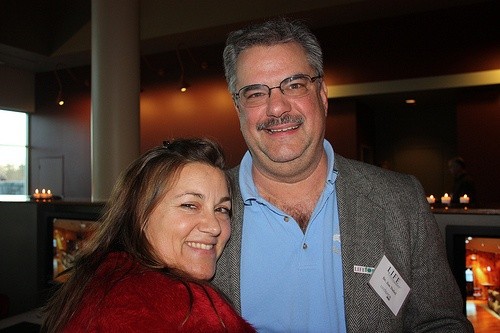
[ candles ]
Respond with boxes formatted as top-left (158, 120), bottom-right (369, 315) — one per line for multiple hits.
top-left (47, 190), bottom-right (53, 202)
top-left (40, 188), bottom-right (47, 202)
top-left (426, 194), bottom-right (436, 203)
top-left (460, 194), bottom-right (470, 204)
top-left (33, 188), bottom-right (40, 202)
top-left (441, 192), bottom-right (452, 203)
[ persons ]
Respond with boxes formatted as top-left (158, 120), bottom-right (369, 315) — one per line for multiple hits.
top-left (37, 138), bottom-right (254, 333)
top-left (206, 21), bottom-right (474, 333)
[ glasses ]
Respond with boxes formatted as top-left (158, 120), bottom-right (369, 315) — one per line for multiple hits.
top-left (233, 75), bottom-right (320, 107)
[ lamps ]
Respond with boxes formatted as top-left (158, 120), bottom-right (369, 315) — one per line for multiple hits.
top-left (55, 70), bottom-right (65, 107)
top-left (174, 44), bottom-right (190, 93)
